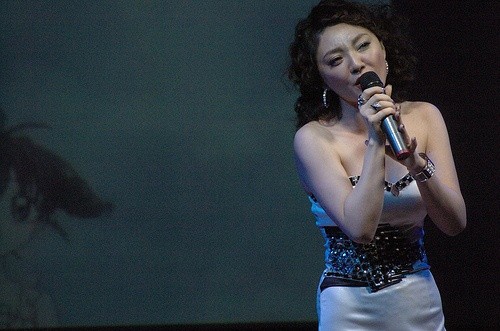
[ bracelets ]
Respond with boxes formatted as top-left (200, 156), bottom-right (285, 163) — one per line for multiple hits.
top-left (406, 152), bottom-right (437, 184)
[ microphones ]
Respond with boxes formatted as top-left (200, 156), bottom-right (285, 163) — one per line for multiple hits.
top-left (360, 73), bottom-right (411, 161)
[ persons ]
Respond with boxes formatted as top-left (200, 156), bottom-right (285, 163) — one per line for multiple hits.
top-left (279, 0), bottom-right (471, 331)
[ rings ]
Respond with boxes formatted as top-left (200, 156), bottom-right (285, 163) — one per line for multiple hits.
top-left (370, 101), bottom-right (381, 112)
top-left (357, 93), bottom-right (365, 107)
